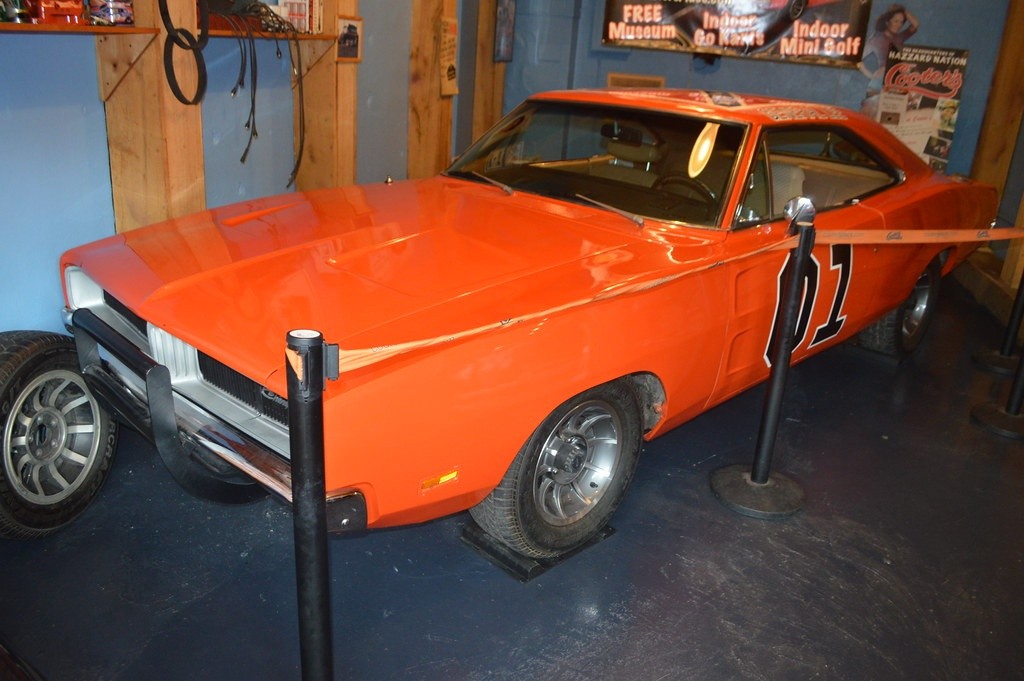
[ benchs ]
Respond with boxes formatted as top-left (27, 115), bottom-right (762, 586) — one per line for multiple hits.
top-left (669, 148), bottom-right (884, 215)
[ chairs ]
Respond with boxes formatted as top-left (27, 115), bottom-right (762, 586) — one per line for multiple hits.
top-left (742, 154), bottom-right (805, 225)
top-left (592, 130), bottom-right (668, 190)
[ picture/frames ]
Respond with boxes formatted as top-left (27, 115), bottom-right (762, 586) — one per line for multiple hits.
top-left (492, 0), bottom-right (516, 63)
top-left (334, 14), bottom-right (364, 63)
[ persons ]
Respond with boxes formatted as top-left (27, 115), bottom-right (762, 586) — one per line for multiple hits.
top-left (859, 5), bottom-right (918, 81)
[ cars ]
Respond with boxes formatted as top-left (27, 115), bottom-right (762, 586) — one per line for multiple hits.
top-left (56, 89), bottom-right (1003, 581)
